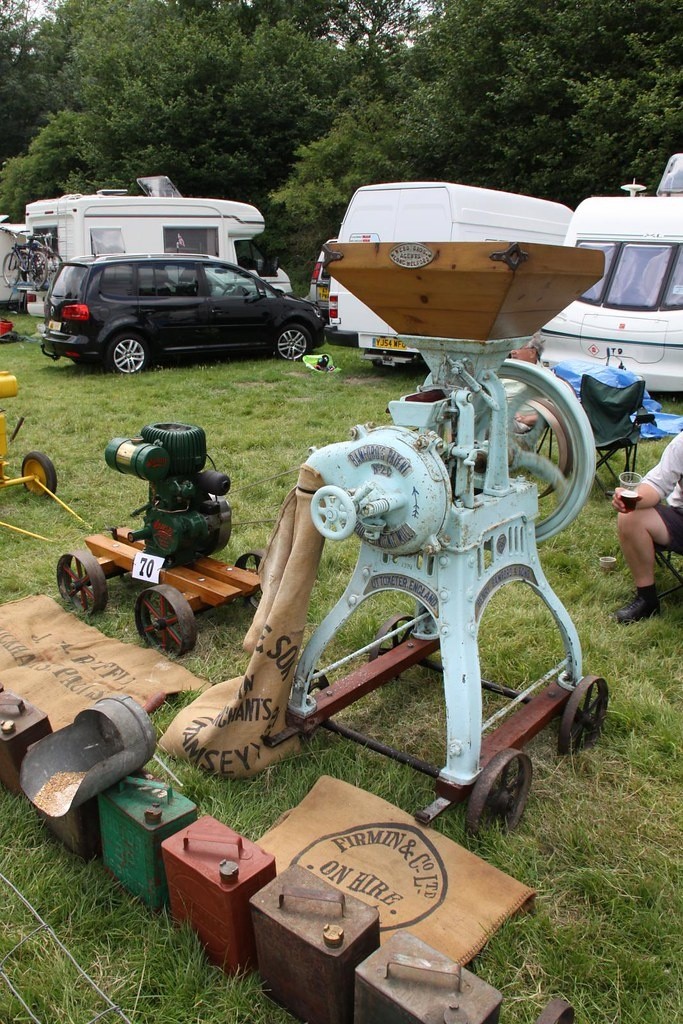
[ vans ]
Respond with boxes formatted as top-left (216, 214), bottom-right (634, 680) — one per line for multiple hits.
top-left (25, 174), bottom-right (293, 317)
top-left (310, 182), bottom-right (576, 375)
top-left (314, 239), bottom-right (336, 321)
top-left (536, 152), bottom-right (683, 396)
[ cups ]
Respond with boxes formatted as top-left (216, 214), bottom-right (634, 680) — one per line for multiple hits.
top-left (619, 473), bottom-right (642, 512)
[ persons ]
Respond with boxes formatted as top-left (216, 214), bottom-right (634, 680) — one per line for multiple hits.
top-left (175, 232), bottom-right (185, 248)
top-left (450, 334), bottom-right (556, 501)
top-left (610, 430), bottom-right (683, 626)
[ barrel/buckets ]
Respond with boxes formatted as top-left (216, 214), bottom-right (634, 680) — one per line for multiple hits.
top-left (0, 320), bottom-right (12, 334)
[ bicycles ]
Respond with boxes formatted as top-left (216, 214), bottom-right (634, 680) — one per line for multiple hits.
top-left (0, 226), bottom-right (64, 291)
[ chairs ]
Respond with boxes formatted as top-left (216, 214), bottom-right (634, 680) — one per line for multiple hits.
top-left (175, 267), bottom-right (198, 295)
top-left (652, 546), bottom-right (683, 600)
top-left (578, 374), bottom-right (656, 497)
top-left (154, 269), bottom-right (173, 295)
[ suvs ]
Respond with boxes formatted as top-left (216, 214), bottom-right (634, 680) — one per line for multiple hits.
top-left (42, 253), bottom-right (329, 376)
